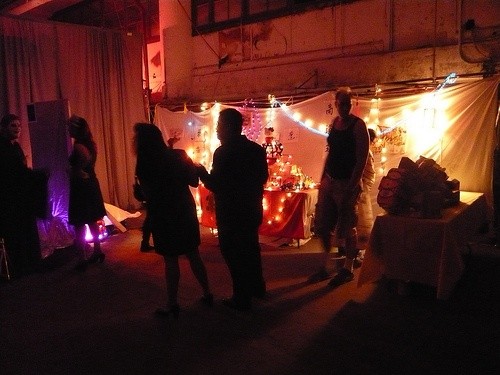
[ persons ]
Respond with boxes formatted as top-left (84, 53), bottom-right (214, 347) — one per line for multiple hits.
top-left (0, 113), bottom-right (49, 280)
top-left (67, 115), bottom-right (107, 273)
top-left (192, 108), bottom-right (268, 312)
top-left (133, 123), bottom-right (214, 318)
top-left (314, 88), bottom-right (370, 288)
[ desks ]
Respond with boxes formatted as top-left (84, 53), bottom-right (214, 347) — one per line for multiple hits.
top-left (358, 190), bottom-right (488, 301)
top-left (198, 184), bottom-right (320, 249)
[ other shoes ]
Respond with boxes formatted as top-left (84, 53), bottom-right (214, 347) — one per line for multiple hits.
top-left (308, 265), bottom-right (331, 284)
top-left (141, 240), bottom-right (154, 251)
top-left (328, 269), bottom-right (354, 286)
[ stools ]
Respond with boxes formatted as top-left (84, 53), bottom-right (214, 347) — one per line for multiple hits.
top-left (467, 241), bottom-right (500, 276)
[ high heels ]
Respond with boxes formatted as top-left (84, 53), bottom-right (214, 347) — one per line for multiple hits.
top-left (87, 252), bottom-right (105, 264)
